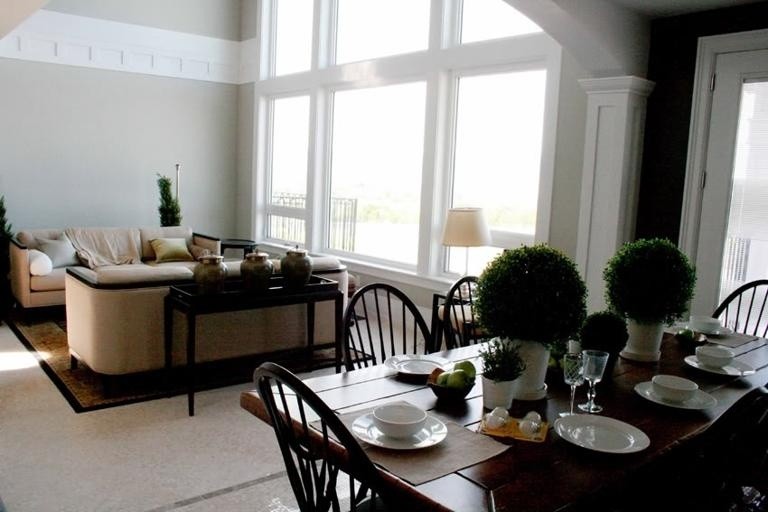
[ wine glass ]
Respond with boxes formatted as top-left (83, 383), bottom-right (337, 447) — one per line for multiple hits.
top-left (561, 351), bottom-right (586, 414)
top-left (576, 350), bottom-right (609, 412)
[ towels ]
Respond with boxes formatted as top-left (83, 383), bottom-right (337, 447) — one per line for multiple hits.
top-left (309, 404), bottom-right (512, 485)
top-left (706, 332), bottom-right (759, 348)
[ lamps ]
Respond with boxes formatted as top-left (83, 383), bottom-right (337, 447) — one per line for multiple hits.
top-left (442, 208), bottom-right (491, 276)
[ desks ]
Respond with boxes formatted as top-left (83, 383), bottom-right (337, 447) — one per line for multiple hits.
top-left (164, 276), bottom-right (344, 416)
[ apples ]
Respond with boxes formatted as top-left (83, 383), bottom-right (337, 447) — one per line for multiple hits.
top-left (548, 356), bottom-right (556, 366)
top-left (437, 360), bottom-right (476, 388)
top-left (559, 355), bottom-right (573, 368)
top-left (678, 329), bottom-right (692, 337)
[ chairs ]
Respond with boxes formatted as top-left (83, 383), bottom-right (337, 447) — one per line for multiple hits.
top-left (341, 284), bottom-right (437, 371)
top-left (253, 362), bottom-right (405, 511)
top-left (443, 276), bottom-right (480, 350)
top-left (430, 293), bottom-right (494, 349)
top-left (712, 279), bottom-right (768, 339)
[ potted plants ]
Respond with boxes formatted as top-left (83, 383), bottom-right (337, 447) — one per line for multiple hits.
top-left (603, 237), bottom-right (696, 361)
top-left (474, 245), bottom-right (587, 400)
top-left (580, 311), bottom-right (629, 380)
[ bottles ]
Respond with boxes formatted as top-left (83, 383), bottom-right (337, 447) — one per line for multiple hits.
top-left (566, 334), bottom-right (581, 361)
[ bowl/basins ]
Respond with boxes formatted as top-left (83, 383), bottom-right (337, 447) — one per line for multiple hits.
top-left (424, 367), bottom-right (475, 399)
top-left (651, 375), bottom-right (697, 405)
top-left (688, 314), bottom-right (720, 335)
top-left (675, 331), bottom-right (708, 351)
top-left (696, 346), bottom-right (735, 368)
top-left (372, 402), bottom-right (426, 441)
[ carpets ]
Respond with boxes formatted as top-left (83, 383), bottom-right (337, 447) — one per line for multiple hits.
top-left (1, 308), bottom-right (374, 413)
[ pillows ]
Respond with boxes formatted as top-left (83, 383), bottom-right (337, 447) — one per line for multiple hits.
top-left (17, 227), bottom-right (211, 276)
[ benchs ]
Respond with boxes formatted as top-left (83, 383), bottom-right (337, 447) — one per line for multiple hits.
top-left (65, 252), bottom-right (359, 399)
top-left (10, 225), bottom-right (212, 326)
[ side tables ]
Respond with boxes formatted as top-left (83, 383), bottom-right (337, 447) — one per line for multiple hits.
top-left (221, 239), bottom-right (257, 260)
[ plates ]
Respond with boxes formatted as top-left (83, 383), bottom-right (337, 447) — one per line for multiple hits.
top-left (631, 379), bottom-right (717, 411)
top-left (682, 353), bottom-right (755, 377)
top-left (384, 353), bottom-right (459, 376)
top-left (351, 412), bottom-right (449, 450)
top-left (552, 413), bottom-right (652, 456)
top-left (676, 321), bottom-right (733, 337)
